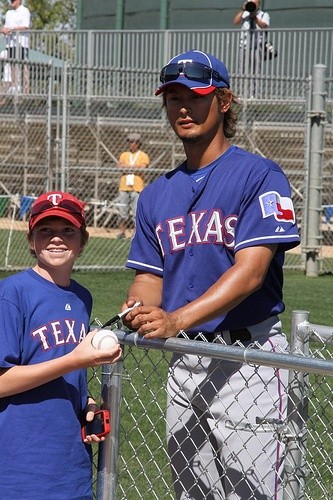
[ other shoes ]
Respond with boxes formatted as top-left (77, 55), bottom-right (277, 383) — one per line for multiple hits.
top-left (117, 233), bottom-right (125, 238)
top-left (7, 86), bottom-right (22, 95)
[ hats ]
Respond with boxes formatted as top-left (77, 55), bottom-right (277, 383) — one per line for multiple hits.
top-left (155, 49), bottom-right (230, 95)
top-left (126, 133), bottom-right (141, 141)
top-left (29, 192), bottom-right (86, 231)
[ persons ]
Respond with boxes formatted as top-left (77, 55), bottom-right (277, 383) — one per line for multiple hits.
top-left (234, 0), bottom-right (270, 98)
top-left (1, 191), bottom-right (121, 500)
top-left (115, 132), bottom-right (152, 238)
top-left (0, 0), bottom-right (34, 95)
top-left (119, 48), bottom-right (301, 500)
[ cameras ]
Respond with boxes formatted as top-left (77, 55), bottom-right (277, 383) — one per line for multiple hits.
top-left (245, 1), bottom-right (256, 12)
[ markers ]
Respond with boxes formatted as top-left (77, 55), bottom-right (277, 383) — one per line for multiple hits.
top-left (101, 301), bottom-right (143, 328)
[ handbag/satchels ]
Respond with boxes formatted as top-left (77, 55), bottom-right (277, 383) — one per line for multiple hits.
top-left (260, 41), bottom-right (277, 61)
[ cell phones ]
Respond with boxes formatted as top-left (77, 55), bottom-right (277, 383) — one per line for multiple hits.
top-left (81, 409), bottom-right (111, 442)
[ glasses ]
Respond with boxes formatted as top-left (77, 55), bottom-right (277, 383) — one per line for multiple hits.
top-left (29, 199), bottom-right (84, 226)
top-left (31, 199), bottom-right (83, 221)
top-left (160, 62), bottom-right (230, 88)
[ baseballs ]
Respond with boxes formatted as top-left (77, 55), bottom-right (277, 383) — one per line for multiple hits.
top-left (92, 330), bottom-right (120, 351)
